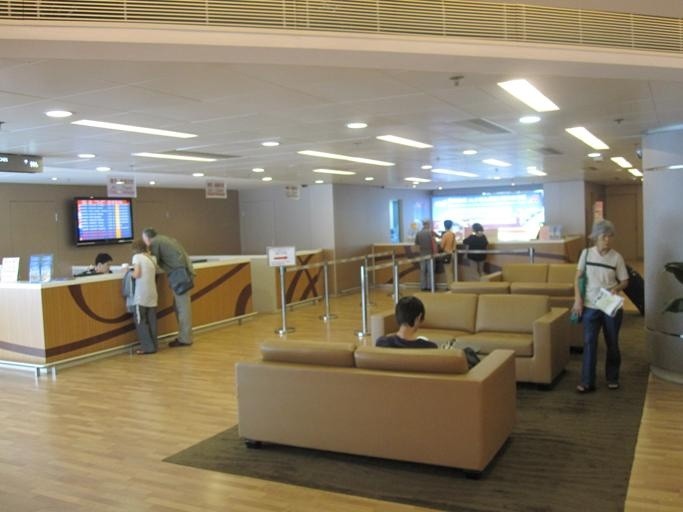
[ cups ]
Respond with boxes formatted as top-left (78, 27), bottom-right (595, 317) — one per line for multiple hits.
top-left (121, 263), bottom-right (129, 269)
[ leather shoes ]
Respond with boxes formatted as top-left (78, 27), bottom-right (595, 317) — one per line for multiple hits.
top-left (135, 349), bottom-right (142, 355)
top-left (168, 339), bottom-right (189, 347)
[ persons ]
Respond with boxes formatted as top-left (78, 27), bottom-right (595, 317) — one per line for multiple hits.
top-left (462, 222), bottom-right (489, 282)
top-left (439, 220), bottom-right (457, 291)
top-left (568, 219), bottom-right (630, 395)
top-left (121, 239), bottom-right (160, 354)
top-left (142, 227), bottom-right (197, 346)
top-left (413, 219), bottom-right (441, 292)
top-left (76, 251), bottom-right (114, 277)
top-left (373, 295), bottom-right (439, 349)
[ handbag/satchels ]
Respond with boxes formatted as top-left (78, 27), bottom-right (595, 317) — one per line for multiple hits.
top-left (436, 249), bottom-right (451, 263)
top-left (167, 267), bottom-right (193, 296)
top-left (570, 273), bottom-right (586, 323)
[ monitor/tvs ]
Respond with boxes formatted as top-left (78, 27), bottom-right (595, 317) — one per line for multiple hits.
top-left (72, 195), bottom-right (136, 247)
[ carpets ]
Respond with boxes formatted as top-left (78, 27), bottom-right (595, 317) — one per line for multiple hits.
top-left (162, 310), bottom-right (652, 511)
